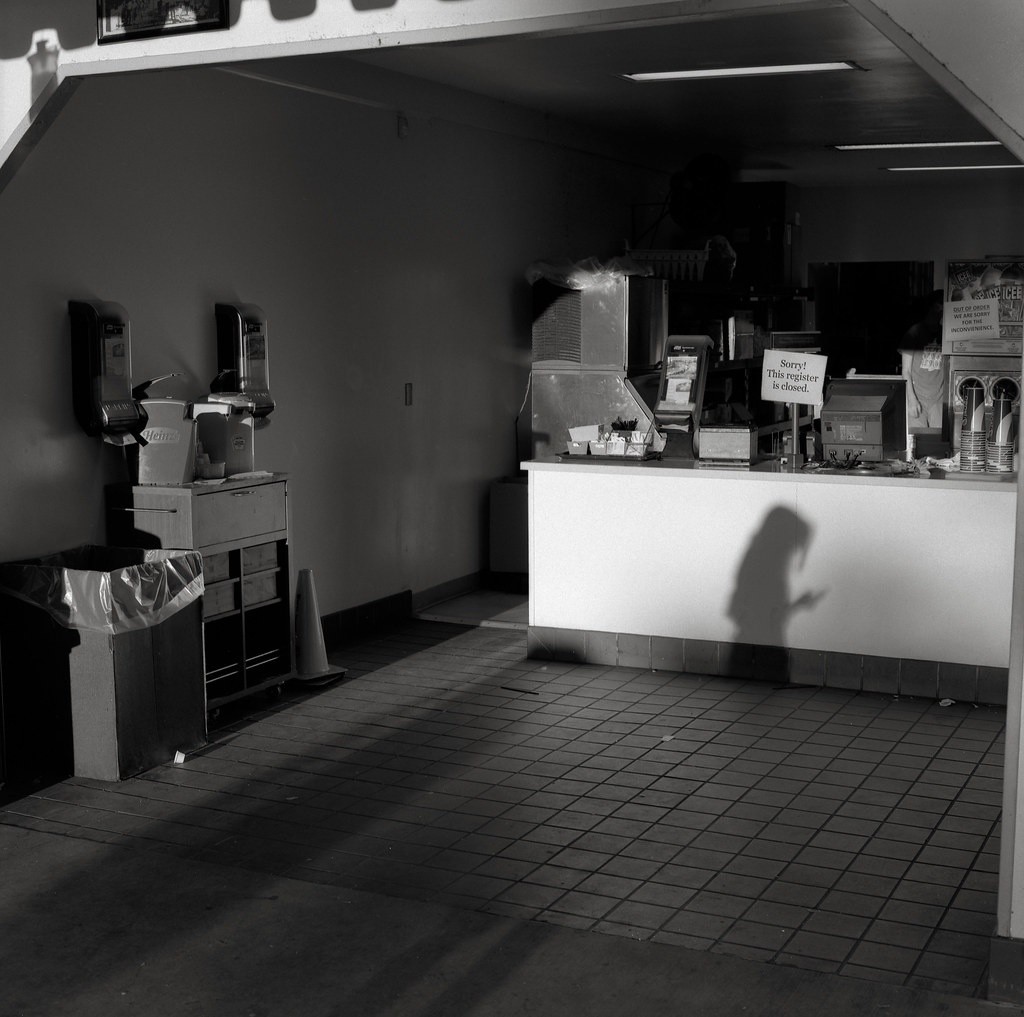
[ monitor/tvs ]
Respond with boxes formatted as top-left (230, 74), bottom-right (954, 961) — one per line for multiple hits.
top-left (820, 379), bottom-right (908, 463)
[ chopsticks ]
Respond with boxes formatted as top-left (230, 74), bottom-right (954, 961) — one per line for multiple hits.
top-left (611, 416), bottom-right (638, 431)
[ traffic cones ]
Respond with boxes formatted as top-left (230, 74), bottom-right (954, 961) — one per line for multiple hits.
top-left (292, 567), bottom-right (348, 690)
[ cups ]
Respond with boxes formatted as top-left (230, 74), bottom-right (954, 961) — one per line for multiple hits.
top-left (961, 388), bottom-right (1016, 476)
top-left (614, 431), bottom-right (634, 437)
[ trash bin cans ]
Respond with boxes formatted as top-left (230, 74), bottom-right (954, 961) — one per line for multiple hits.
top-left (6, 545), bottom-right (209, 783)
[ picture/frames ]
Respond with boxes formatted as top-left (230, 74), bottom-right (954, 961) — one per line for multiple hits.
top-left (97, 0), bottom-right (230, 45)
